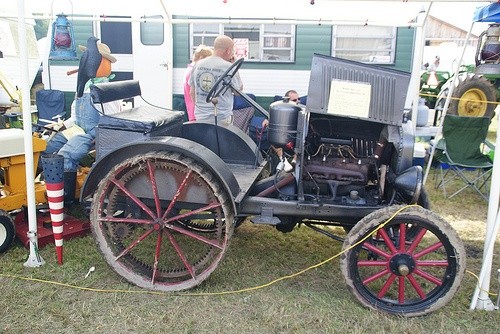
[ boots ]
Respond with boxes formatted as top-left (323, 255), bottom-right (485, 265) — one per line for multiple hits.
top-left (63, 171), bottom-right (77, 207)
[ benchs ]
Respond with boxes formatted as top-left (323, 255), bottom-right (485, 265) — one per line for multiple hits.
top-left (88, 79), bottom-right (186, 135)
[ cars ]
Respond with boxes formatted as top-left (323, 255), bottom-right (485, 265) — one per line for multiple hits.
top-left (79, 49), bottom-right (466, 319)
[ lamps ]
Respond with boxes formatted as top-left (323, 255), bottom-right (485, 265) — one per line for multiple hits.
top-left (47, 13), bottom-right (79, 61)
top-left (474, 22), bottom-right (500, 79)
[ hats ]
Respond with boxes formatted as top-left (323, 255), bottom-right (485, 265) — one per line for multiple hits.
top-left (77, 42), bottom-right (117, 63)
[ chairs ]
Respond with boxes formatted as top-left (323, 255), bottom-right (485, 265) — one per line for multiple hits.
top-left (34, 90), bottom-right (65, 130)
top-left (434, 114), bottom-right (494, 203)
top-left (235, 94), bottom-right (306, 148)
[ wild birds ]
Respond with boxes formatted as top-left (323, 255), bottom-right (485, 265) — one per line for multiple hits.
top-left (76, 36), bottom-right (102, 98)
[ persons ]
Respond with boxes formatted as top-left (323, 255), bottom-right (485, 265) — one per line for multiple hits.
top-left (184, 46), bottom-right (213, 121)
top-left (188, 35), bottom-right (243, 125)
top-left (35, 43), bottom-right (123, 207)
top-left (262, 90), bottom-right (300, 171)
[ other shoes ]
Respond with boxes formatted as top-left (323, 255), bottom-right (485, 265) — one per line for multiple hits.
top-left (276, 157), bottom-right (296, 172)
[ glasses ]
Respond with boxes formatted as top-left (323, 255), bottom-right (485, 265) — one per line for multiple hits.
top-left (292, 98), bottom-right (300, 102)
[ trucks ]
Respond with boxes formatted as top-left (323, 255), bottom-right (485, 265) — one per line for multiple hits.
top-left (0, 0), bottom-right (419, 156)
